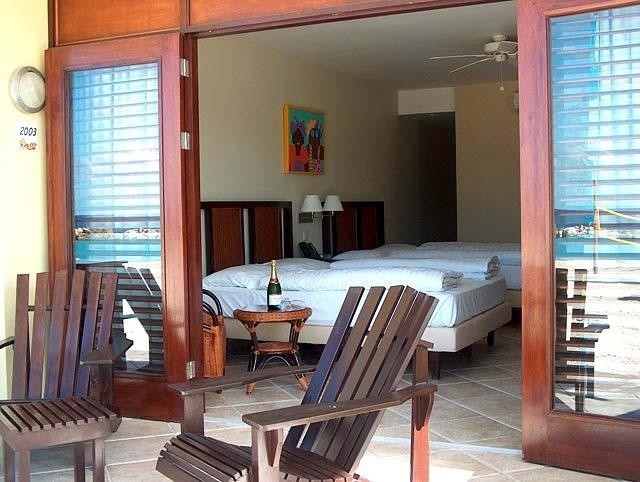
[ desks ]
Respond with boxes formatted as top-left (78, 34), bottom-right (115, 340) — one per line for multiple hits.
top-left (233, 301), bottom-right (311, 394)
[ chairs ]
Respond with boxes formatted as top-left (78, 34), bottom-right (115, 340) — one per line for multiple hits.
top-left (153, 282), bottom-right (439, 481)
top-left (74, 260), bottom-right (166, 373)
top-left (2, 268), bottom-right (133, 482)
top-left (556, 269), bottom-right (608, 415)
top-left (202, 288), bottom-right (228, 395)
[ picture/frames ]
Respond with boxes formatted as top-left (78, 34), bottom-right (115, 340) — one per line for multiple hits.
top-left (281, 103), bottom-right (331, 179)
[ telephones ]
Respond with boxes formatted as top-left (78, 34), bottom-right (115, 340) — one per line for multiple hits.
top-left (299, 242), bottom-right (320, 258)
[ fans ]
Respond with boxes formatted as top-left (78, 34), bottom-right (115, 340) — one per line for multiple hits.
top-left (427, 34), bottom-right (520, 76)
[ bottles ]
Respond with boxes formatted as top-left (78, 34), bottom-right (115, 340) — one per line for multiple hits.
top-left (267, 260), bottom-right (282, 310)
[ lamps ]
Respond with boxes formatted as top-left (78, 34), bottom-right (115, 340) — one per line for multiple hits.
top-left (513, 90), bottom-right (519, 113)
top-left (497, 58), bottom-right (507, 94)
top-left (301, 193), bottom-right (344, 261)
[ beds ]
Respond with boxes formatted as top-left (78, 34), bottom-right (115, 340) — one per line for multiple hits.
top-left (323, 203), bottom-right (522, 324)
top-left (202, 202), bottom-right (513, 367)
